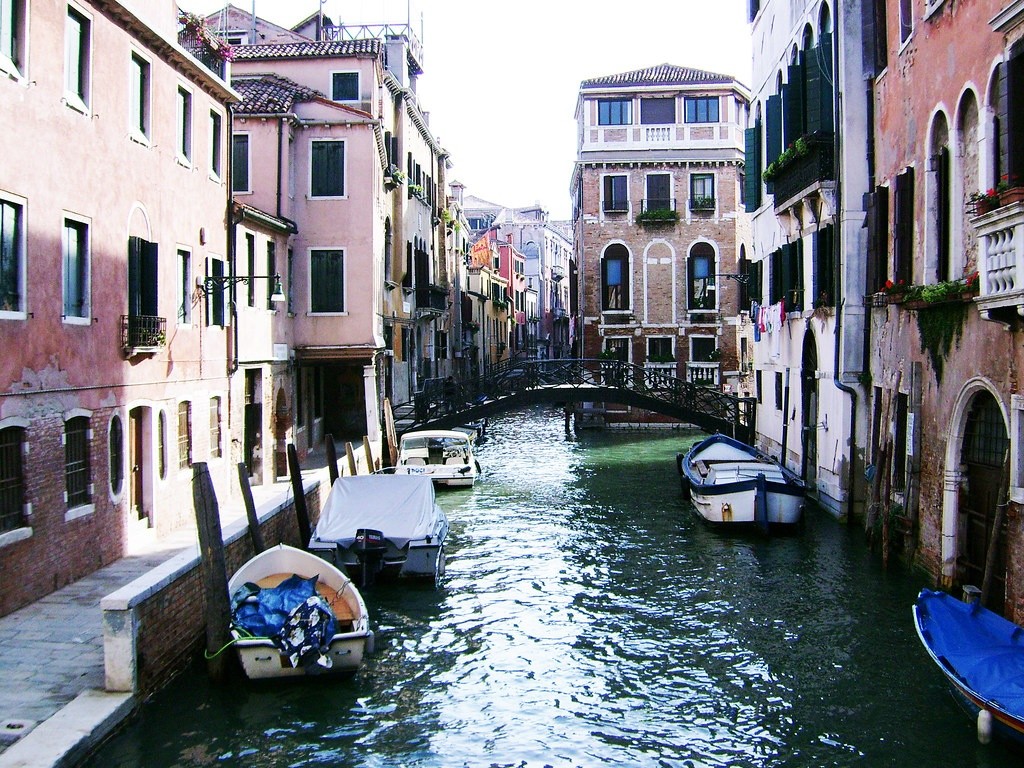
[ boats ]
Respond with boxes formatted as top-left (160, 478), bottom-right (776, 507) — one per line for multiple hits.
top-left (910, 586), bottom-right (1023, 752)
top-left (677, 428), bottom-right (809, 536)
top-left (224, 543), bottom-right (377, 681)
top-left (306, 473), bottom-right (451, 589)
top-left (390, 418), bottom-right (490, 485)
